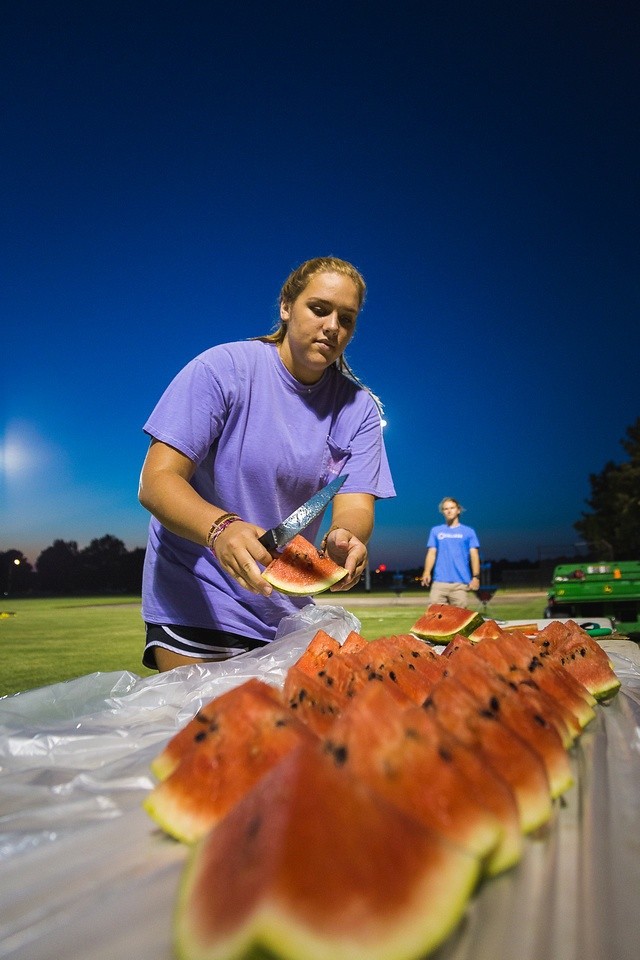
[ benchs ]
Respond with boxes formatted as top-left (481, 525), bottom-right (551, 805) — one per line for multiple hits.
top-left (544, 561), bottom-right (640, 618)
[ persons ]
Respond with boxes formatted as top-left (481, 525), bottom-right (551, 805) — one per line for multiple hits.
top-left (421, 497), bottom-right (480, 608)
top-left (138, 256), bottom-right (396, 673)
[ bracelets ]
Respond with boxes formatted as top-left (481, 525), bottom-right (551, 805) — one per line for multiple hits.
top-left (207, 513), bottom-right (245, 558)
top-left (472, 574), bottom-right (480, 580)
top-left (322, 526), bottom-right (353, 553)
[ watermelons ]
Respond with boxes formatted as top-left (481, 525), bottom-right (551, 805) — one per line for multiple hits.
top-left (260, 534), bottom-right (349, 596)
top-left (141, 603), bottom-right (622, 960)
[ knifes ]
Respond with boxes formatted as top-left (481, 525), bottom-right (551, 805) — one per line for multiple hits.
top-left (255, 475), bottom-right (349, 553)
